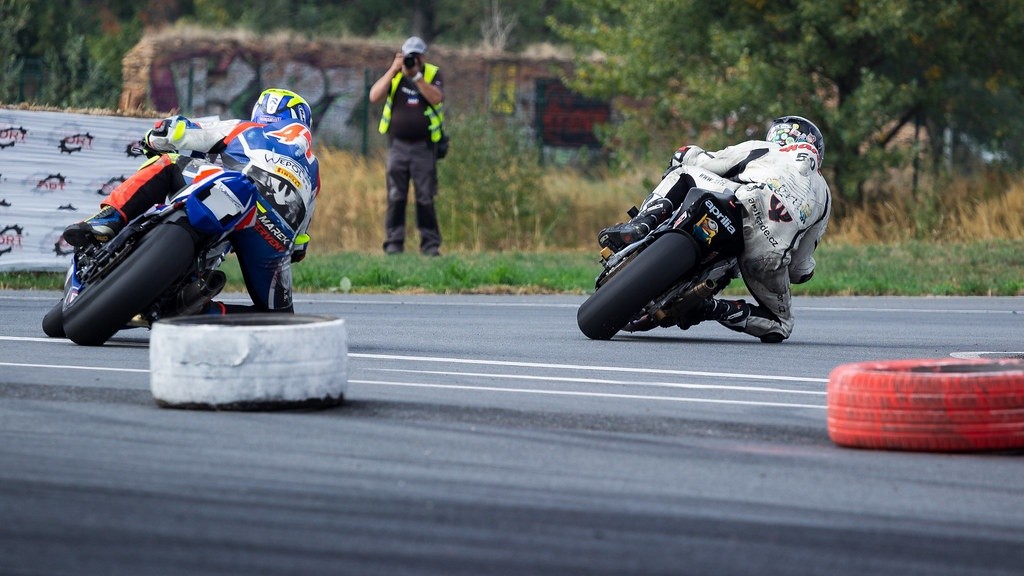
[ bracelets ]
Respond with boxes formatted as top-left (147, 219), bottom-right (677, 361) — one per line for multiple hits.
top-left (412, 72), bottom-right (422, 82)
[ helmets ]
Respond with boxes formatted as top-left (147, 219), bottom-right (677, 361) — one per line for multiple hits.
top-left (766, 115), bottom-right (824, 169)
top-left (251, 88), bottom-right (313, 129)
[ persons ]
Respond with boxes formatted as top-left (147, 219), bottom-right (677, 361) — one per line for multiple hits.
top-left (62, 88), bottom-right (321, 315)
top-left (597, 116), bottom-right (831, 344)
top-left (369, 36), bottom-right (444, 256)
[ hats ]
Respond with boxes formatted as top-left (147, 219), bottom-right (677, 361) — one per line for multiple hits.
top-left (402, 36), bottom-right (427, 57)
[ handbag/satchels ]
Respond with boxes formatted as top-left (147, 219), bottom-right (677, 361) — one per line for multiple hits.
top-left (435, 135), bottom-right (448, 158)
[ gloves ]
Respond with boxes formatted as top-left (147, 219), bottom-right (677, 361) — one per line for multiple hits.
top-left (672, 145), bottom-right (695, 167)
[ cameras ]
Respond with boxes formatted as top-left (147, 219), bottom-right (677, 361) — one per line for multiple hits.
top-left (405, 54), bottom-right (415, 69)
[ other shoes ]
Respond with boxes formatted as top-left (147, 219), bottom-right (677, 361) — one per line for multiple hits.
top-left (425, 247), bottom-right (439, 257)
top-left (385, 243), bottom-right (401, 253)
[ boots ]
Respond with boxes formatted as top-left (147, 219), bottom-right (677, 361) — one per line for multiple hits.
top-left (678, 299), bottom-right (750, 333)
top-left (598, 198), bottom-right (673, 254)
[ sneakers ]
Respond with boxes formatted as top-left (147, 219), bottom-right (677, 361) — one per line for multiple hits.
top-left (63, 206), bottom-right (123, 248)
top-left (201, 300), bottom-right (222, 315)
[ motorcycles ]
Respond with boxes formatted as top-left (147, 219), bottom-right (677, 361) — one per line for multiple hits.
top-left (577, 164), bottom-right (753, 340)
top-left (40, 137), bottom-right (254, 347)
